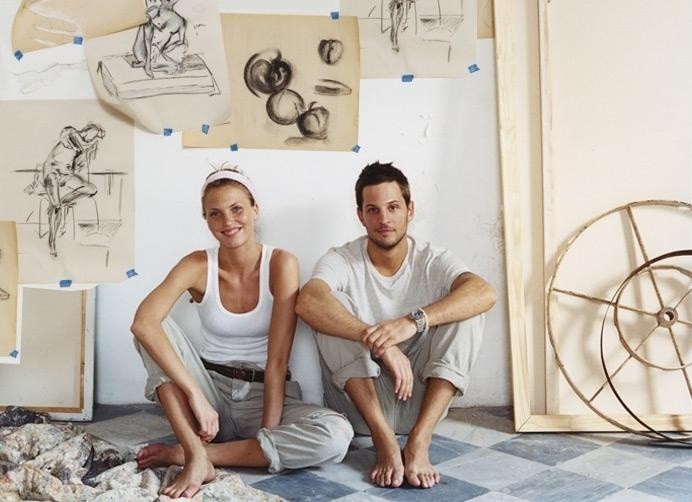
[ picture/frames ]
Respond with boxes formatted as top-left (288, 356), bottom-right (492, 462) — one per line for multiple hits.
top-left (492, 0), bottom-right (691, 434)
top-left (0, 285), bottom-right (96, 423)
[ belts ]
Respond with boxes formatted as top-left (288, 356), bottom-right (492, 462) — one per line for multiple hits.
top-left (199, 356), bottom-right (291, 383)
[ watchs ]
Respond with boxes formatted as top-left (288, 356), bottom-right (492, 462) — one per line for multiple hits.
top-left (408, 309), bottom-right (425, 333)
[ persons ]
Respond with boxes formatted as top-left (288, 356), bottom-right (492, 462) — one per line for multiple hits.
top-left (294, 159), bottom-right (498, 489)
top-left (128, 167), bottom-right (354, 500)
